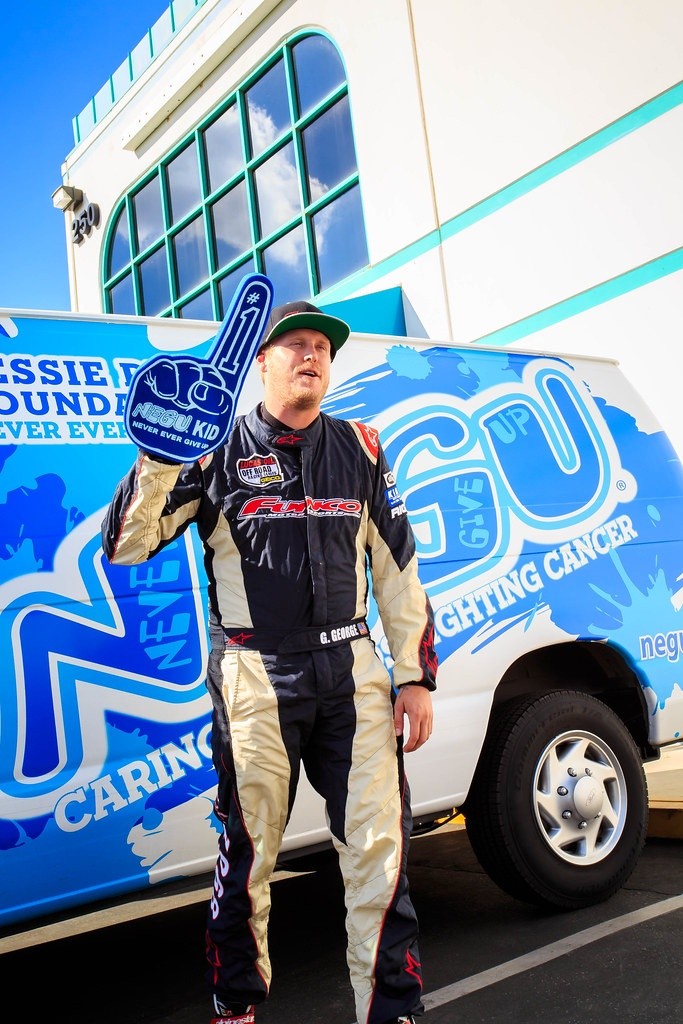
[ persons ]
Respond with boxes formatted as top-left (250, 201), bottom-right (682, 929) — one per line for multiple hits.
top-left (102, 300), bottom-right (438, 1023)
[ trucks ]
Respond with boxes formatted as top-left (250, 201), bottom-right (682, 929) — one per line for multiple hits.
top-left (0, 308), bottom-right (683, 955)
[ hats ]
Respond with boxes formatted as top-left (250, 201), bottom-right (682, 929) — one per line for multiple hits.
top-left (255, 300), bottom-right (351, 364)
